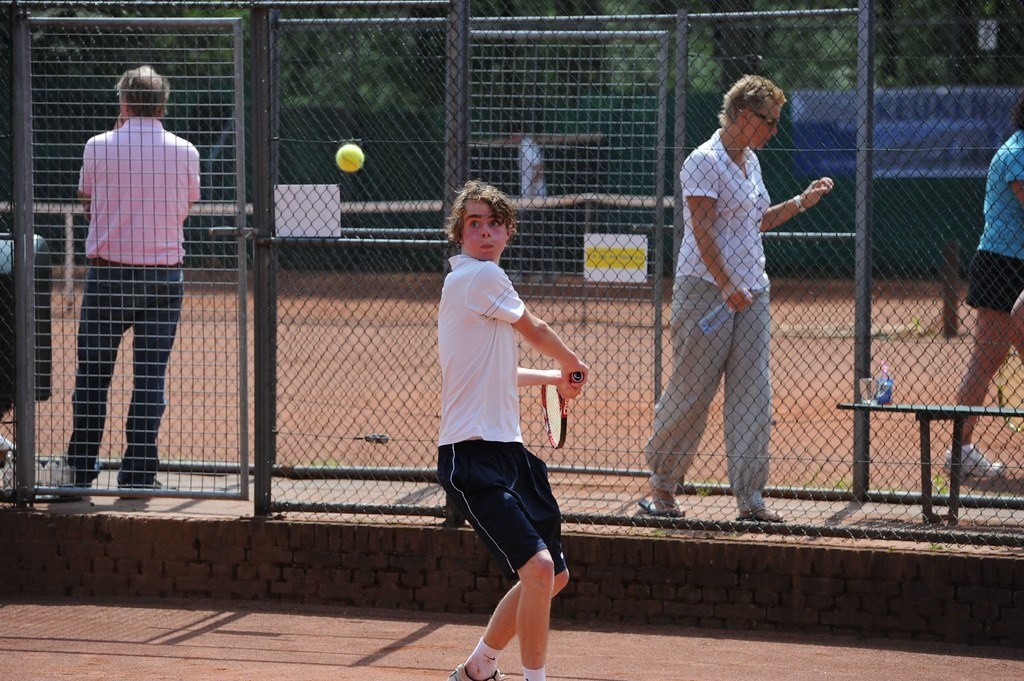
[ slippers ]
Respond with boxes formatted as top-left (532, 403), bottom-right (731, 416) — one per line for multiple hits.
top-left (736, 508), bottom-right (786, 523)
top-left (638, 498), bottom-right (684, 516)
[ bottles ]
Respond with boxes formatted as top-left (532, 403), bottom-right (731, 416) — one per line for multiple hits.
top-left (698, 289), bottom-right (752, 335)
top-left (878, 358), bottom-right (893, 406)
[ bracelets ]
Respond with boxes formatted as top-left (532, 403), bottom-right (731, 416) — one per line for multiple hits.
top-left (791, 194), bottom-right (805, 213)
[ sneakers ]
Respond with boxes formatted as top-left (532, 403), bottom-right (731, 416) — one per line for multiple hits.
top-left (448, 665), bottom-right (501, 681)
top-left (942, 450), bottom-right (1005, 480)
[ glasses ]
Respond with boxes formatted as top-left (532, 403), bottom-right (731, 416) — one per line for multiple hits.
top-left (753, 112), bottom-right (782, 128)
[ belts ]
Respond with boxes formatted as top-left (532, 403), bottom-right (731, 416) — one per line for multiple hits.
top-left (90, 255), bottom-right (184, 269)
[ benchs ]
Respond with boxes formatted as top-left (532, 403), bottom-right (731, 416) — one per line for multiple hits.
top-left (835, 401), bottom-right (1024, 527)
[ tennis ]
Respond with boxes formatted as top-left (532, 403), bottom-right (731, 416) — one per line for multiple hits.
top-left (336, 144), bottom-right (365, 172)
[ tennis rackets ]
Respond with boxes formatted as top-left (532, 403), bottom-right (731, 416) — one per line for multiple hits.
top-left (541, 370), bottom-right (584, 450)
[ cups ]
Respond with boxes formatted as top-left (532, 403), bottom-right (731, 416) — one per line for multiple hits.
top-left (860, 376), bottom-right (873, 404)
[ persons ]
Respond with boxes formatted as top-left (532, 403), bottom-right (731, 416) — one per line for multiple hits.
top-left (49, 61), bottom-right (202, 501)
top-left (1, 194), bottom-right (50, 469)
top-left (436, 177), bottom-right (590, 681)
top-left (939, 87), bottom-right (1024, 487)
top-left (507, 116), bottom-right (571, 286)
top-left (642, 73), bottom-right (837, 524)
